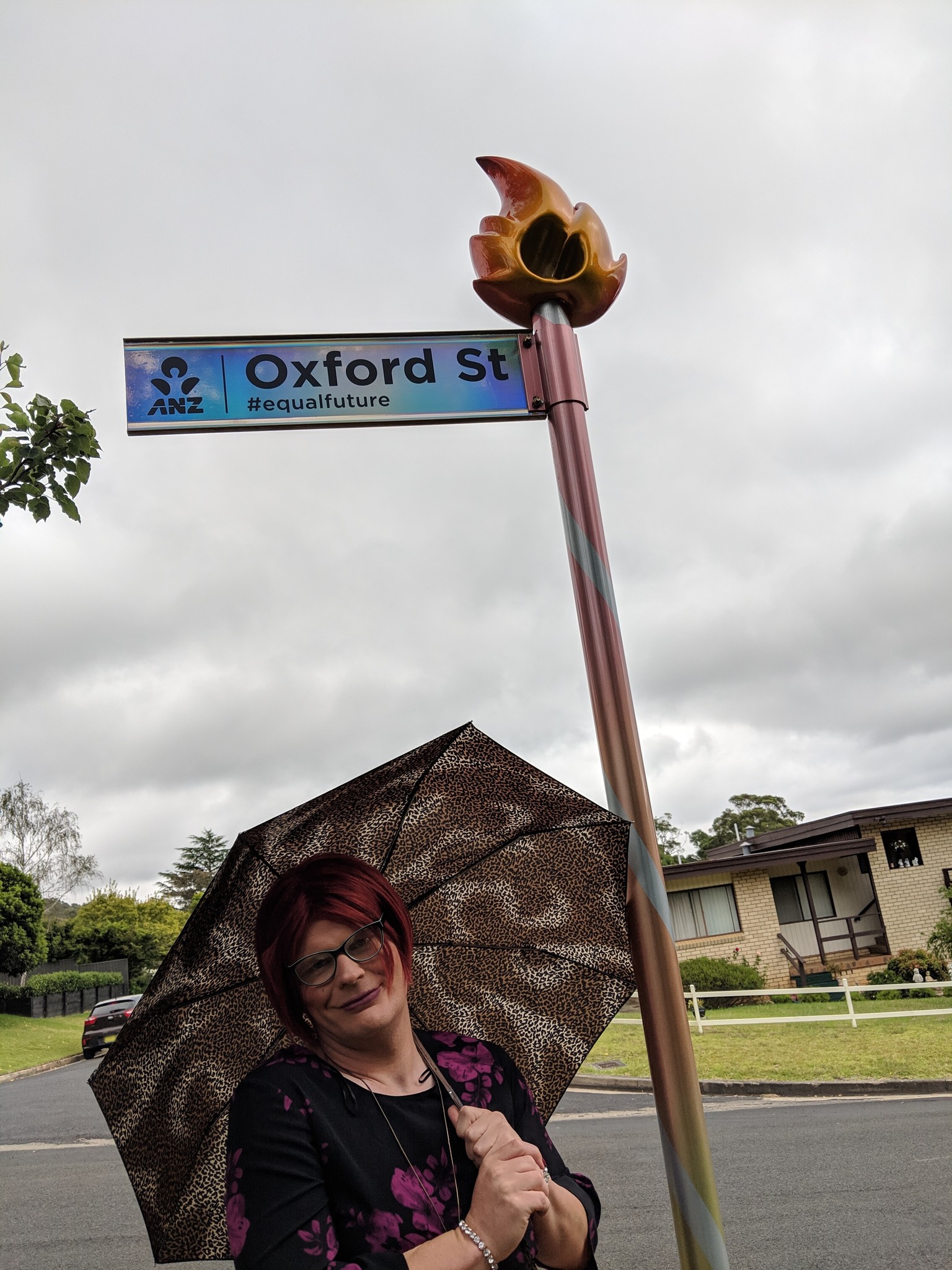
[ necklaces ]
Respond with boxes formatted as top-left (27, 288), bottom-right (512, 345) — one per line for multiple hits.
top-left (330, 1046), bottom-right (465, 1238)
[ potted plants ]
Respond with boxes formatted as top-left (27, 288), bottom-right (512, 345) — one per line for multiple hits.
top-left (822, 958), bottom-right (843, 987)
top-left (688, 998), bottom-right (706, 1018)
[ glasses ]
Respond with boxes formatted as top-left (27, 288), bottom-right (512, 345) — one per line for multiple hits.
top-left (284, 911), bottom-right (385, 987)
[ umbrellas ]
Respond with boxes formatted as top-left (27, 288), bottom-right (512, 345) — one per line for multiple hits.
top-left (83, 714), bottom-right (647, 1268)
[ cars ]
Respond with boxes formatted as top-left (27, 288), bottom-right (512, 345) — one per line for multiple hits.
top-left (81, 993), bottom-right (144, 1061)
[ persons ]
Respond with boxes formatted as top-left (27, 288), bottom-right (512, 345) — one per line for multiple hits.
top-left (216, 849), bottom-right (607, 1269)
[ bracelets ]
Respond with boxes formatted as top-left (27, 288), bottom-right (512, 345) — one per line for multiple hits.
top-left (543, 1165), bottom-right (552, 1187)
top-left (455, 1215), bottom-right (501, 1270)
